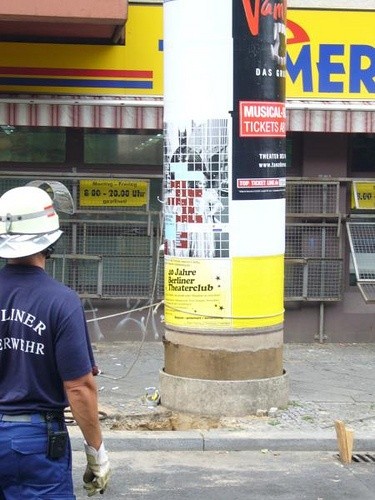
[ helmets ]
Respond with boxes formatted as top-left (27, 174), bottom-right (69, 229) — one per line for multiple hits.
top-left (1, 179), bottom-right (74, 259)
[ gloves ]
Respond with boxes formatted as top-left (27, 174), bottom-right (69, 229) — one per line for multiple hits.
top-left (80, 444), bottom-right (112, 496)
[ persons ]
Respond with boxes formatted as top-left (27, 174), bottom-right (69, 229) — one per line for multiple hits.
top-left (0, 186), bottom-right (112, 500)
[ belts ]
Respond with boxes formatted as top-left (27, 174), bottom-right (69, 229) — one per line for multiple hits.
top-left (1, 413), bottom-right (64, 424)
